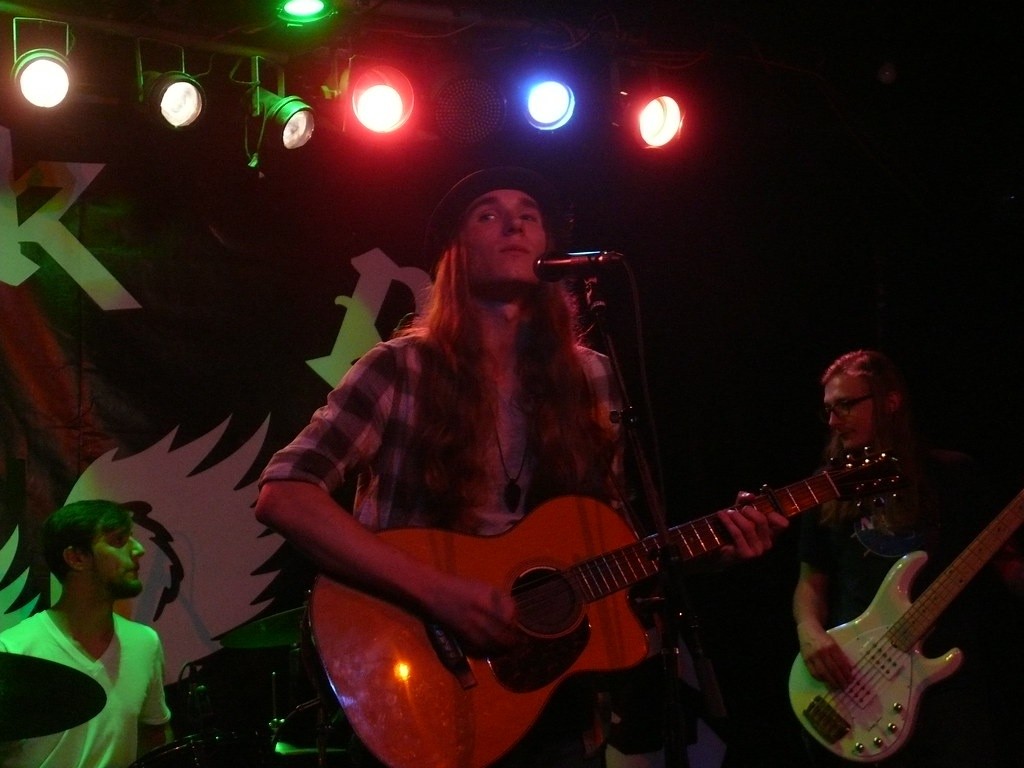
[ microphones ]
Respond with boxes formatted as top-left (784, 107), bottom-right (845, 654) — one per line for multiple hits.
top-left (532, 249), bottom-right (623, 283)
top-left (185, 664), bottom-right (199, 724)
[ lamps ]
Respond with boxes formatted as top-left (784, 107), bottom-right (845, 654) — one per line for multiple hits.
top-left (607, 59), bottom-right (683, 147)
top-left (430, 62), bottom-right (509, 147)
top-left (320, 40), bottom-right (413, 135)
top-left (519, 68), bottom-right (577, 132)
top-left (9, 17), bottom-right (79, 113)
top-left (127, 37), bottom-right (207, 132)
top-left (227, 55), bottom-right (316, 149)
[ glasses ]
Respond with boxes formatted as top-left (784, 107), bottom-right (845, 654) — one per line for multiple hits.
top-left (820, 394), bottom-right (876, 419)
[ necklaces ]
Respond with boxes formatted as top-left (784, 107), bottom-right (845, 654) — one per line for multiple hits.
top-left (488, 386), bottom-right (535, 514)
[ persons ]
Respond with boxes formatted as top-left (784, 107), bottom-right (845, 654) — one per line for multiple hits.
top-left (0, 500), bottom-right (170, 768)
top-left (257, 173), bottom-right (790, 768)
top-left (792, 350), bottom-right (1024, 768)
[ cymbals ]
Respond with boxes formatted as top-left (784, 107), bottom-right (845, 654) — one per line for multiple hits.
top-left (218, 604), bottom-right (312, 653)
top-left (0, 650), bottom-right (108, 744)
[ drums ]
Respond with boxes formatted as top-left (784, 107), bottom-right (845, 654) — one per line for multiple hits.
top-left (125, 719), bottom-right (274, 768)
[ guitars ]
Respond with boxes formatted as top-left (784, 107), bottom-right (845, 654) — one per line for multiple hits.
top-left (310, 445), bottom-right (913, 768)
top-left (787, 488), bottom-right (1024, 768)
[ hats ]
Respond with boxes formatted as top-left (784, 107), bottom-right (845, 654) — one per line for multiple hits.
top-left (422, 165), bottom-right (562, 276)
